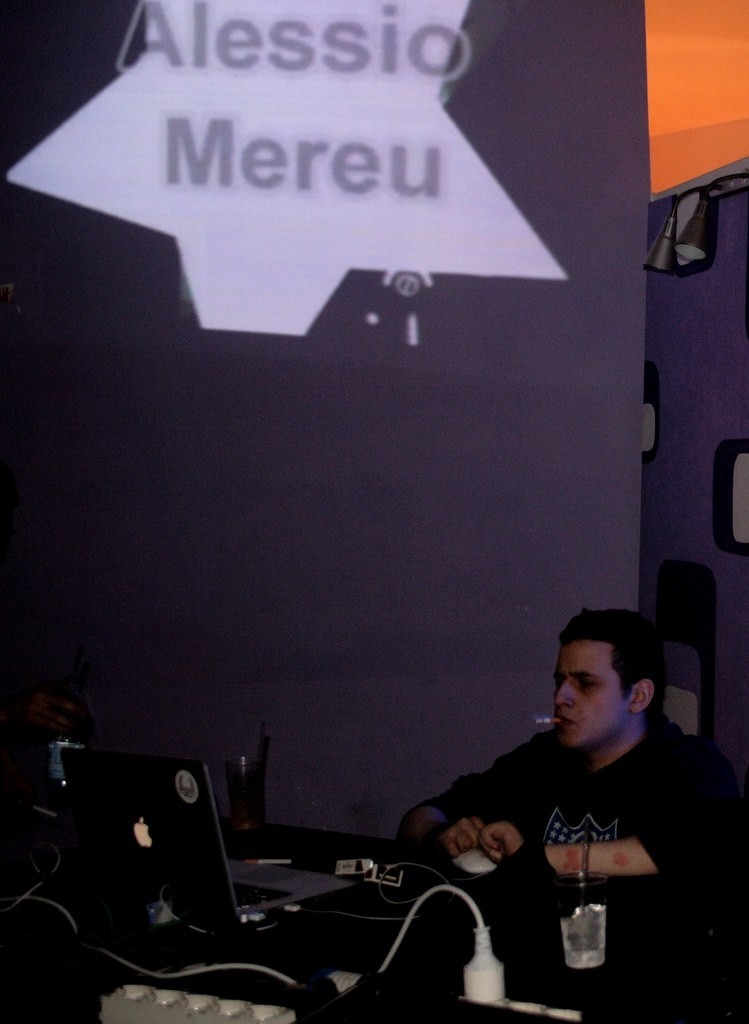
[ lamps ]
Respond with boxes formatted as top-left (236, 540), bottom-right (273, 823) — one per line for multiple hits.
top-left (672, 173), bottom-right (749, 260)
top-left (642, 184), bottom-right (721, 277)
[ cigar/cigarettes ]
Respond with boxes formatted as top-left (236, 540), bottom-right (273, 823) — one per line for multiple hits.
top-left (535, 715), bottom-right (561, 726)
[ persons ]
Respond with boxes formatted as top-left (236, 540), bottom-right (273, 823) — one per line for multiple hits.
top-left (395, 606), bottom-right (741, 879)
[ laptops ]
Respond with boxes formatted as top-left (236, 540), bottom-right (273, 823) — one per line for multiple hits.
top-left (60, 746), bottom-right (363, 919)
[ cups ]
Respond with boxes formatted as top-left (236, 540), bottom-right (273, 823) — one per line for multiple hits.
top-left (554, 871), bottom-right (609, 969)
top-left (224, 757), bottom-right (266, 832)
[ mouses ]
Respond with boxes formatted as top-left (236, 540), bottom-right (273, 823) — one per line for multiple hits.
top-left (453, 848), bottom-right (497, 874)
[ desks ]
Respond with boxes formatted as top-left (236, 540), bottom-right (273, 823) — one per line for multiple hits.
top-left (0, 817), bottom-right (749, 1024)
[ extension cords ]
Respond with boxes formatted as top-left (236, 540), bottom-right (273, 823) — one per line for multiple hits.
top-left (462, 994), bottom-right (580, 1023)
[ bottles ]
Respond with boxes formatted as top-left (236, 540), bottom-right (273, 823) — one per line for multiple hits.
top-left (45, 677), bottom-right (89, 806)
